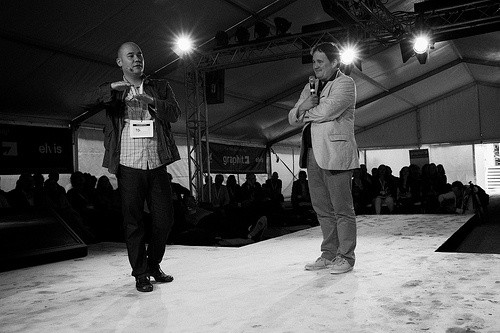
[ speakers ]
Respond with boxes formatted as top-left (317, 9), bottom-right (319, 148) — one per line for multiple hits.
top-left (0, 206), bottom-right (88, 272)
top-left (204, 68), bottom-right (225, 105)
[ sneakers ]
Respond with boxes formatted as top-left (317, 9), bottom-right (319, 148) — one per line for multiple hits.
top-left (305, 256), bottom-right (331, 271)
top-left (330, 256), bottom-right (353, 273)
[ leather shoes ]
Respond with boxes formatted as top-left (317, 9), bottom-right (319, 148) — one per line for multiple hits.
top-left (148, 266), bottom-right (173, 282)
top-left (136, 276), bottom-right (153, 292)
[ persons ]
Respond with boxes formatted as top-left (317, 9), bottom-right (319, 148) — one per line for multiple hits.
top-left (78, 41), bottom-right (182, 292)
top-left (0, 164), bottom-right (490, 243)
top-left (288, 42), bottom-right (360, 274)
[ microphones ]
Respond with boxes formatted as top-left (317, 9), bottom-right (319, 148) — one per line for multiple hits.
top-left (125, 68), bottom-right (131, 70)
top-left (309, 76), bottom-right (316, 95)
